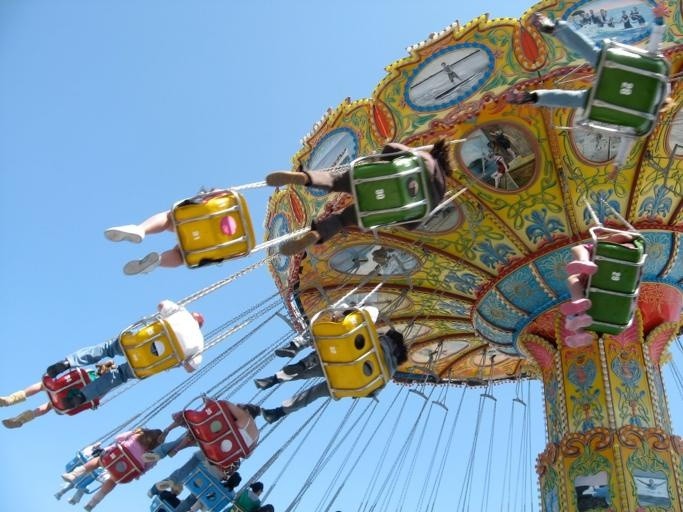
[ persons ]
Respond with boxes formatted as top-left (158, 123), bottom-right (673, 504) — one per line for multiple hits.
top-left (273, 299), bottom-right (379, 376)
top-left (52, 398), bottom-right (275, 512)
top-left (503, 10), bottom-right (601, 109)
top-left (263, 135), bottom-right (454, 257)
top-left (0, 359), bottom-right (117, 431)
top-left (563, 309), bottom-right (594, 349)
top-left (488, 152), bottom-right (510, 189)
top-left (45, 298), bottom-right (205, 407)
top-left (486, 140), bottom-right (514, 162)
top-left (99, 188), bottom-right (221, 276)
top-left (251, 326), bottom-right (409, 424)
top-left (636, 478), bottom-right (669, 490)
top-left (439, 61), bottom-right (462, 85)
top-left (558, 227), bottom-right (638, 315)
top-left (571, 6), bottom-right (646, 31)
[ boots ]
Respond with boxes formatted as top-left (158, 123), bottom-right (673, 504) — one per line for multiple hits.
top-left (253, 376), bottom-right (277, 390)
top-left (261, 407), bottom-right (284, 423)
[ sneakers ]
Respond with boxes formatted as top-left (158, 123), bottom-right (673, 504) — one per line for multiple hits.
top-left (168, 450), bottom-right (176, 457)
top-left (54, 493), bottom-right (60, 499)
top-left (279, 231), bottom-right (321, 257)
top-left (47, 359), bottom-right (69, 378)
top-left (531, 12), bottom-right (554, 32)
top-left (158, 433), bottom-right (165, 443)
top-left (560, 298), bottom-right (592, 348)
top-left (103, 225), bottom-right (145, 243)
top-left (84, 501), bottom-right (94, 511)
top-left (275, 347), bottom-right (295, 357)
top-left (61, 474), bottom-right (75, 482)
top-left (266, 172), bottom-right (308, 186)
top-left (69, 394), bottom-right (83, 404)
top-left (68, 496), bottom-right (78, 504)
top-left (566, 260), bottom-right (597, 275)
top-left (147, 481), bottom-right (182, 498)
top-left (2, 418), bottom-right (23, 428)
top-left (502, 91), bottom-right (529, 104)
top-left (283, 360), bottom-right (303, 374)
top-left (0, 396), bottom-right (14, 406)
top-left (123, 252), bottom-right (161, 275)
